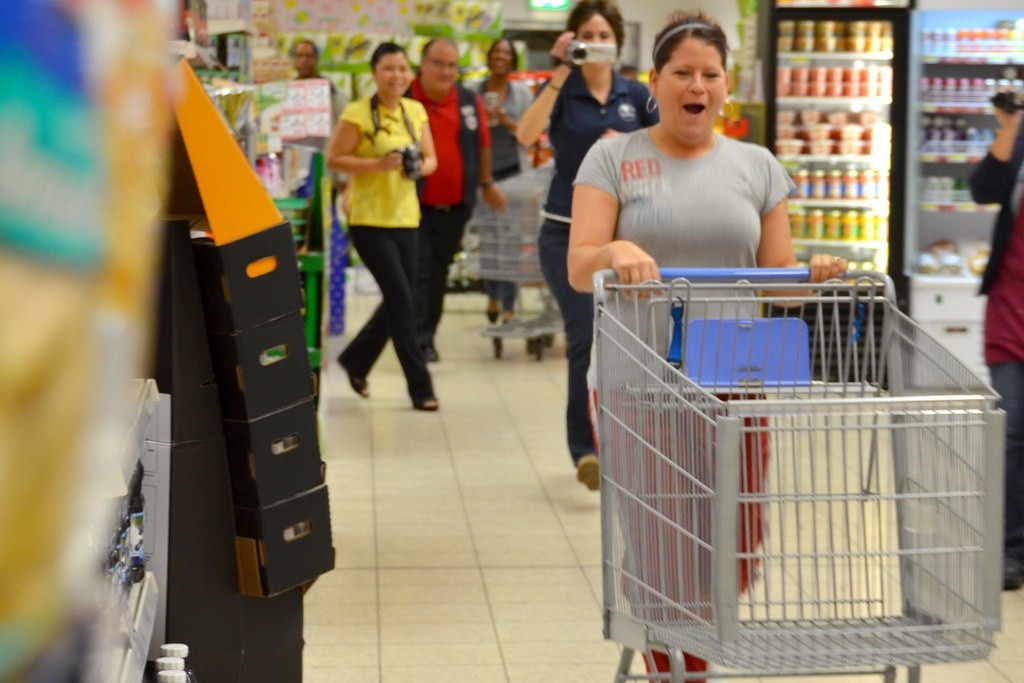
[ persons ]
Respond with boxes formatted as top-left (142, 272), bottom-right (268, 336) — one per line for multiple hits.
top-left (405, 37), bottom-right (509, 362)
top-left (326, 43), bottom-right (441, 411)
top-left (566, 11), bottom-right (848, 683)
top-left (293, 40), bottom-right (338, 124)
top-left (515, 1), bottom-right (659, 490)
top-left (462, 37), bottom-right (535, 328)
top-left (968, 83), bottom-right (1024, 592)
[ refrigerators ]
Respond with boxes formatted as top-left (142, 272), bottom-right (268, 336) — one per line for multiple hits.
top-left (759, 1), bottom-right (908, 385)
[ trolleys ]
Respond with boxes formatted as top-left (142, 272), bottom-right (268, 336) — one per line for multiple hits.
top-left (461, 154), bottom-right (566, 363)
top-left (582, 264), bottom-right (1008, 683)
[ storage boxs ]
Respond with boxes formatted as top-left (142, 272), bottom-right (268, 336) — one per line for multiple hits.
top-left (191, 220), bottom-right (337, 597)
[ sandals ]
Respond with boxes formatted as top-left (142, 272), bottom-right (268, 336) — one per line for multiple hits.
top-left (412, 393), bottom-right (438, 411)
top-left (337, 354), bottom-right (369, 398)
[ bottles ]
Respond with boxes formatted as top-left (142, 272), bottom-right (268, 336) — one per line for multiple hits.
top-left (236, 123), bottom-right (293, 203)
top-left (779, 168), bottom-right (888, 243)
top-left (104, 456), bottom-right (197, 683)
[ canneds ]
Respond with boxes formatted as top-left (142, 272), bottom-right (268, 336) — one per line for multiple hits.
top-left (255, 153), bottom-right (281, 199)
top-left (784, 165), bottom-right (886, 242)
top-left (922, 76), bottom-right (1024, 104)
top-left (920, 28), bottom-right (1024, 53)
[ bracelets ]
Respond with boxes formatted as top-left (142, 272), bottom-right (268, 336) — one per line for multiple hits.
top-left (475, 176), bottom-right (495, 190)
top-left (548, 83), bottom-right (562, 92)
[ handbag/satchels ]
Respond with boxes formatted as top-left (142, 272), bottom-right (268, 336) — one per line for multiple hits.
top-left (483, 113), bottom-right (520, 181)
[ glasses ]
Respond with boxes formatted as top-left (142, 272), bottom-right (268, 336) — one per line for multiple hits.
top-left (425, 57), bottom-right (459, 73)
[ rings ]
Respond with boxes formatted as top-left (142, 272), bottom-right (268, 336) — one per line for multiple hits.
top-left (834, 256), bottom-right (840, 261)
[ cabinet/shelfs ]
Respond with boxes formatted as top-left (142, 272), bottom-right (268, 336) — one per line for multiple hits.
top-left (916, 16), bottom-right (1024, 274)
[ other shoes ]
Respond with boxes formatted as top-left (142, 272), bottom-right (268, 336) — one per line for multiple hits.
top-left (502, 310), bottom-right (513, 324)
top-left (488, 297), bottom-right (499, 324)
top-left (1002, 555), bottom-right (1024, 590)
top-left (420, 342), bottom-right (438, 362)
top-left (577, 453), bottom-right (604, 491)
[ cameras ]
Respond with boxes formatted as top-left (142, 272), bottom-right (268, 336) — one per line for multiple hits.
top-left (566, 40), bottom-right (617, 65)
top-left (990, 92), bottom-right (1024, 115)
top-left (393, 145), bottom-right (423, 179)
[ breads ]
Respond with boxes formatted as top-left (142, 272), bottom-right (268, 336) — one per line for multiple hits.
top-left (916, 239), bottom-right (992, 274)
